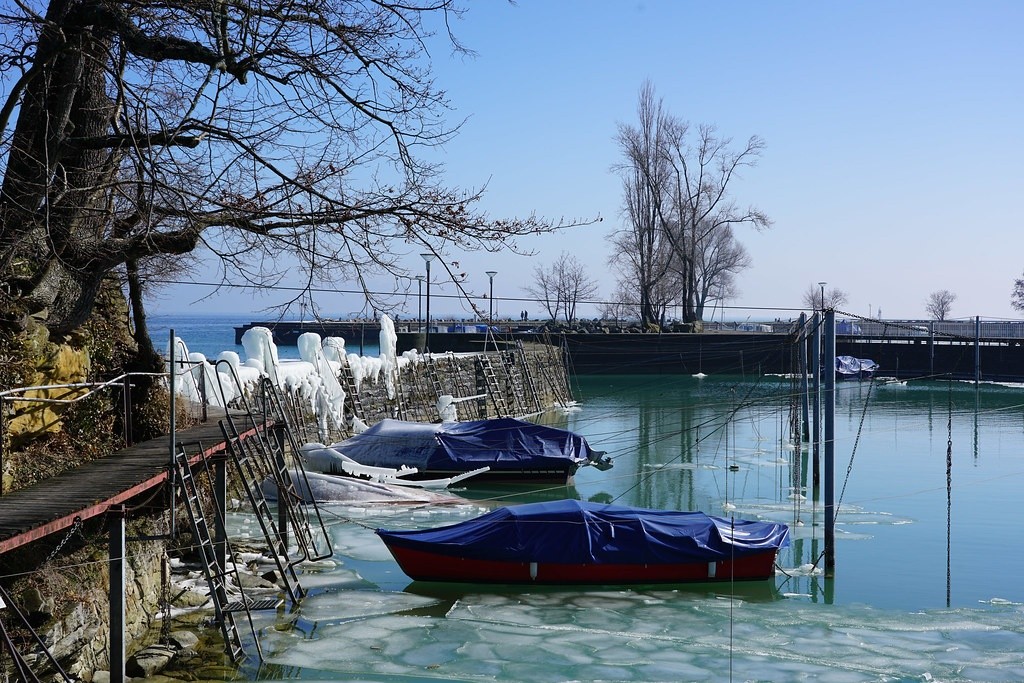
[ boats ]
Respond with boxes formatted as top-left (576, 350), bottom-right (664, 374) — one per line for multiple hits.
top-left (835, 356), bottom-right (880, 380)
top-left (305, 417), bottom-right (590, 485)
top-left (375, 499), bottom-right (791, 585)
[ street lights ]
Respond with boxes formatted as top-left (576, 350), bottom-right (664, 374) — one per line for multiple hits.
top-left (420, 250), bottom-right (436, 353)
top-left (416, 274), bottom-right (425, 333)
top-left (485, 271), bottom-right (497, 327)
top-left (818, 282), bottom-right (827, 320)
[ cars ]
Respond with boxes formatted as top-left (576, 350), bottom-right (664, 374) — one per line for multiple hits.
top-left (836, 324), bottom-right (862, 334)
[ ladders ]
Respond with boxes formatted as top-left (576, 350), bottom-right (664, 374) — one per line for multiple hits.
top-left (260, 342), bottom-right (587, 460)
top-left (170, 417), bottom-right (322, 672)
